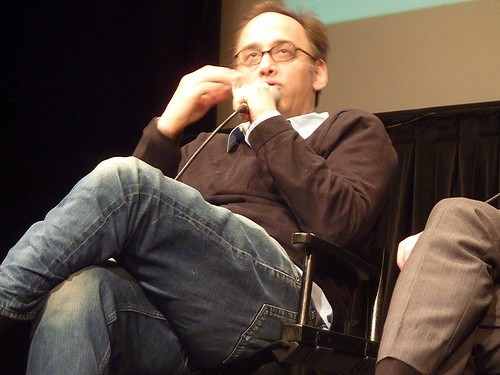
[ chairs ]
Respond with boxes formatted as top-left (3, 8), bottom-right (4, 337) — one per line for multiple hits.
top-left (220, 118), bottom-right (418, 375)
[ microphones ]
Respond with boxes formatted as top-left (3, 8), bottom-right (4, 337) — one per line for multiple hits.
top-left (237, 86), bottom-right (280, 113)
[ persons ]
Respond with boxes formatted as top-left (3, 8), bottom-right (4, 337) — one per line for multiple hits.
top-left (375, 196), bottom-right (500, 375)
top-left (0, 0), bottom-right (398, 375)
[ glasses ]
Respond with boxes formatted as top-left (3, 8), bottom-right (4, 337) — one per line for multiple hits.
top-left (233, 43), bottom-right (317, 67)
